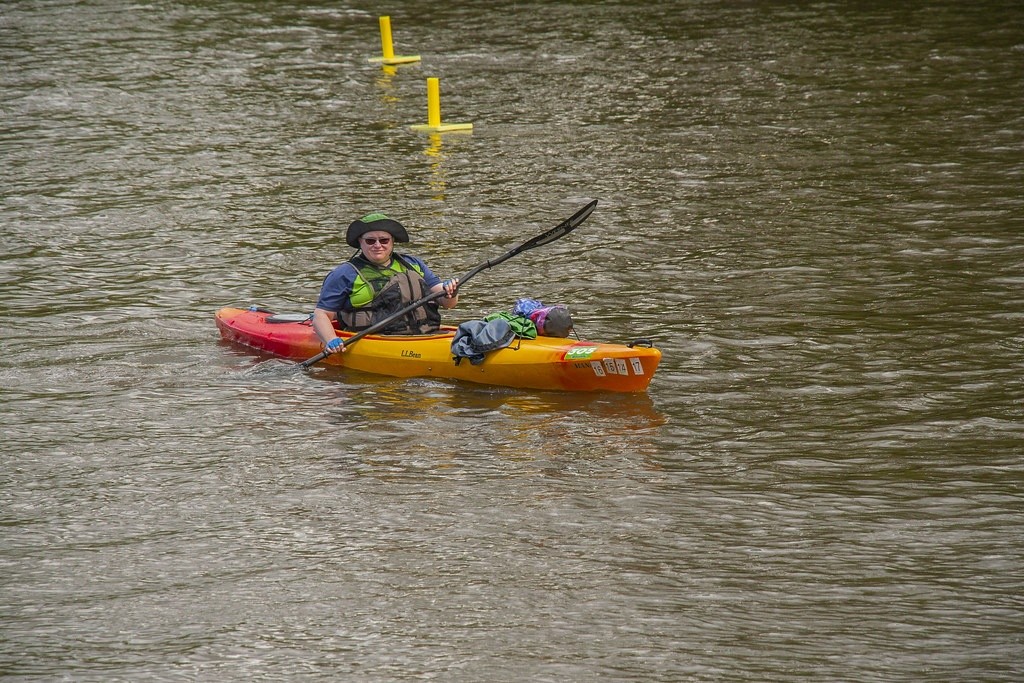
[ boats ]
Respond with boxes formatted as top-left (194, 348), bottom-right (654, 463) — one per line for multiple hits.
top-left (215, 305), bottom-right (661, 394)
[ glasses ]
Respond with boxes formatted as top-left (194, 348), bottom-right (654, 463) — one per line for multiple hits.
top-left (361, 237), bottom-right (391, 245)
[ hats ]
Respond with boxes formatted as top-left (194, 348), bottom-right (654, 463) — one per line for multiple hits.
top-left (345, 214), bottom-right (409, 248)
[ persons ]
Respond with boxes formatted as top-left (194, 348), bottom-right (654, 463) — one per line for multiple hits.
top-left (312, 212), bottom-right (459, 354)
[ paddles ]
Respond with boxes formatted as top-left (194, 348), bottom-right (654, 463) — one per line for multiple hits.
top-left (301, 198), bottom-right (599, 368)
